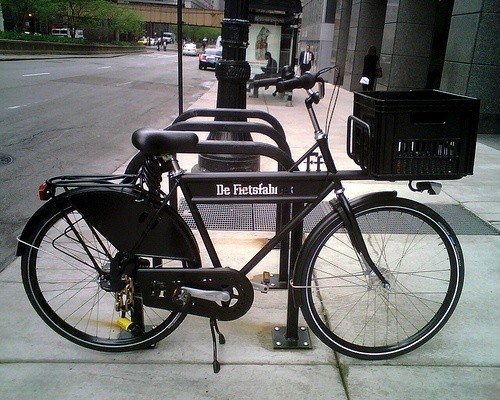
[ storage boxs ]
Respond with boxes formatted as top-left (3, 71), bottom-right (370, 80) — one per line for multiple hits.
top-left (352, 89), bottom-right (481, 176)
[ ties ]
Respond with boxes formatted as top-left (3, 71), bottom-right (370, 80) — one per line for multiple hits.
top-left (305, 53), bottom-right (307, 65)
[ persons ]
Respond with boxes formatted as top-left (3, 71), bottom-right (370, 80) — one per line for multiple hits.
top-left (162, 39), bottom-right (171, 51)
top-left (360, 45), bottom-right (383, 91)
top-left (246, 52), bottom-right (277, 98)
top-left (200, 43), bottom-right (207, 51)
top-left (299, 45), bottom-right (314, 76)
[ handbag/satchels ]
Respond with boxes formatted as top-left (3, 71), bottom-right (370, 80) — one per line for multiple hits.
top-left (376, 56), bottom-right (382, 78)
top-left (359, 77), bottom-right (369, 85)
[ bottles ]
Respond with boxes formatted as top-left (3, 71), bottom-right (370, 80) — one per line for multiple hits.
top-left (396, 140), bottom-right (458, 175)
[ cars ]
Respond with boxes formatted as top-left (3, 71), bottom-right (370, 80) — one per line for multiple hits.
top-left (144, 38), bottom-right (159, 46)
top-left (199, 49), bottom-right (222, 69)
top-left (183, 43), bottom-right (197, 56)
top-left (137, 38), bottom-right (147, 45)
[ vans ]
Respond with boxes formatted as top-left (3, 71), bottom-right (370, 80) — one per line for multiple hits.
top-left (159, 32), bottom-right (175, 44)
top-left (25, 31), bottom-right (42, 36)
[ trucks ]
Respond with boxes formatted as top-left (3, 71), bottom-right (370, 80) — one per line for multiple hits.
top-left (216, 36), bottom-right (222, 50)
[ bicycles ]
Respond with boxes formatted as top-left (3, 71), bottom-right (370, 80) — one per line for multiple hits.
top-left (14, 65), bottom-right (481, 373)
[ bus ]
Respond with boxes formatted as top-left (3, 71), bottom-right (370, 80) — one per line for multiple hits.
top-left (50, 28), bottom-right (83, 39)
